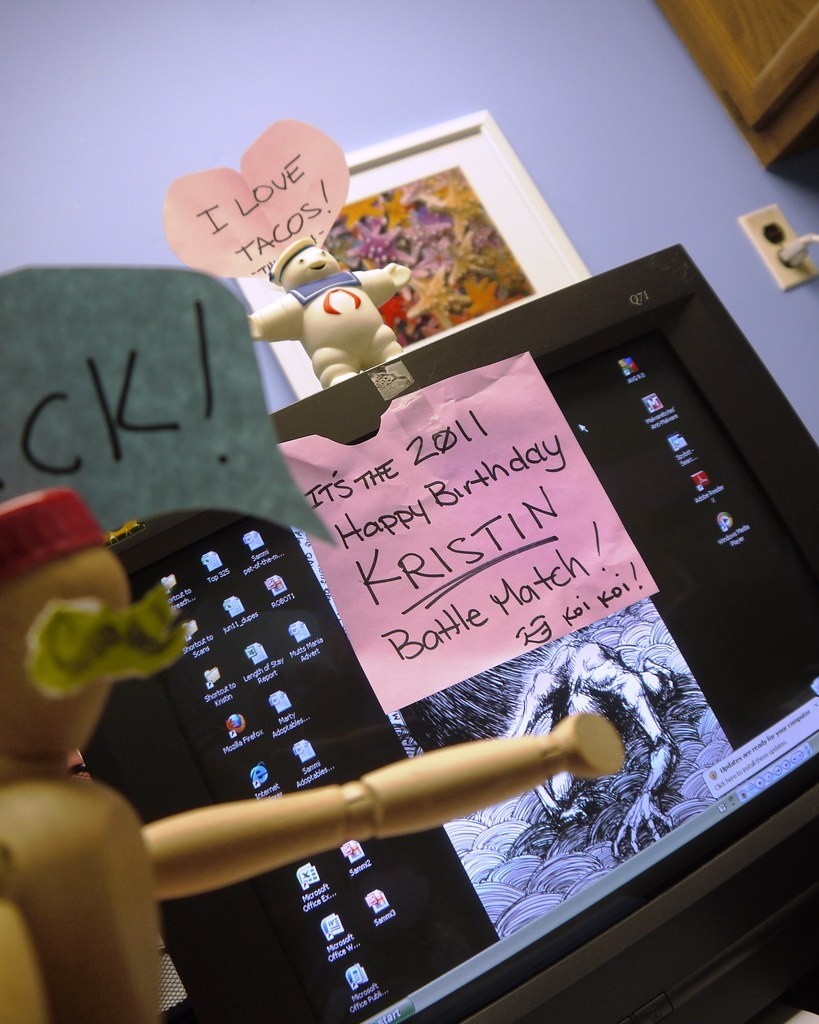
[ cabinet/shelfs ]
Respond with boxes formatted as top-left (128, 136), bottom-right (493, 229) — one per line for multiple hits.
top-left (657, 0), bottom-right (819, 170)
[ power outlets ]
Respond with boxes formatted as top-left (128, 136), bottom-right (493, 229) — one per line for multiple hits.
top-left (738, 203), bottom-right (819, 292)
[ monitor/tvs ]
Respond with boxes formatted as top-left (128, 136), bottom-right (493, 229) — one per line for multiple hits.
top-left (75, 242), bottom-right (819, 1024)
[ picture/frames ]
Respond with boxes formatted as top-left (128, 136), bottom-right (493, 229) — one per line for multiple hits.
top-left (223, 111), bottom-right (592, 398)
